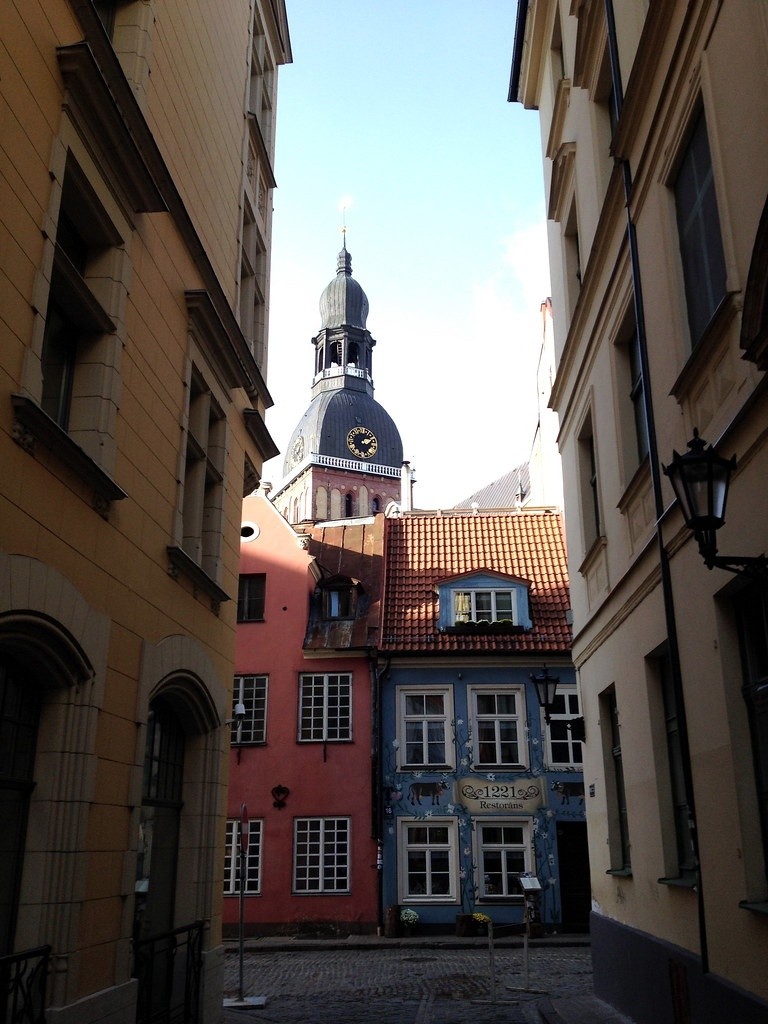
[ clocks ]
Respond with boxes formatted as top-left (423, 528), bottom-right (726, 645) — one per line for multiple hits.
top-left (290, 437), bottom-right (304, 468)
top-left (347, 427), bottom-right (378, 459)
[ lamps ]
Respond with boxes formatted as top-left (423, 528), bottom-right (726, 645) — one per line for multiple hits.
top-left (529, 662), bottom-right (586, 743)
top-left (662, 426), bottom-right (768, 577)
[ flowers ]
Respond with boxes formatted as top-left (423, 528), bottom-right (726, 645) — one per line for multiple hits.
top-left (400, 909), bottom-right (418, 927)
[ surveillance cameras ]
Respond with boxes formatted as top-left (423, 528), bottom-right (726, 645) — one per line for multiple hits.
top-left (234, 704), bottom-right (246, 721)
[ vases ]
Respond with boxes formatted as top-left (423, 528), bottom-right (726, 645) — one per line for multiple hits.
top-left (393, 924), bottom-right (440, 938)
